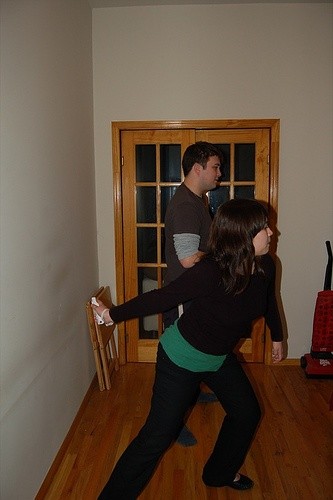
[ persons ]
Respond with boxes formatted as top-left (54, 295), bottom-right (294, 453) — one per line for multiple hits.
top-left (90, 197), bottom-right (285, 500)
top-left (161, 141), bottom-right (221, 448)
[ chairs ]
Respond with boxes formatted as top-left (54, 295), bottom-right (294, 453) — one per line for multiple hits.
top-left (86, 286), bottom-right (117, 392)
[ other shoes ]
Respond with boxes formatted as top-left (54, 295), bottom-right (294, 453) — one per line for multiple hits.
top-left (229, 474), bottom-right (254, 490)
top-left (177, 426), bottom-right (197, 447)
top-left (199, 392), bottom-right (218, 404)
